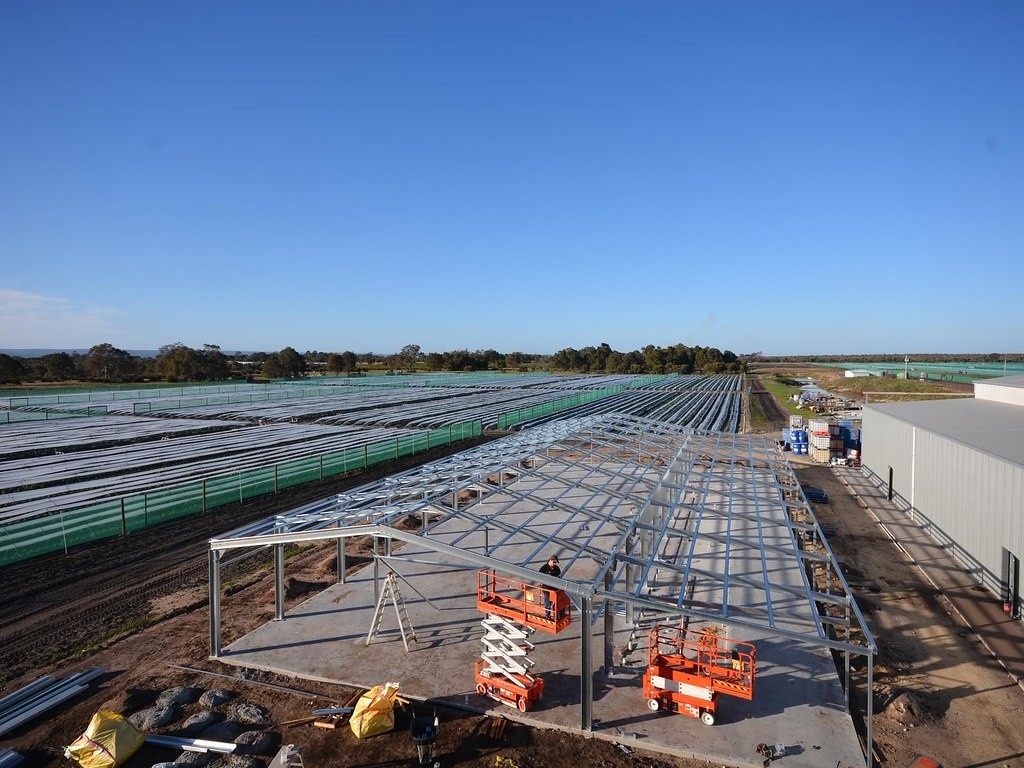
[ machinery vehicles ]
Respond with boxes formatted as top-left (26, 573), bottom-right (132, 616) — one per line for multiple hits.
top-left (641, 623), bottom-right (756, 726)
top-left (475, 570), bottom-right (574, 713)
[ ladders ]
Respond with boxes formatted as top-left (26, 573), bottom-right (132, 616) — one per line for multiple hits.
top-left (365, 571), bottom-right (418, 653)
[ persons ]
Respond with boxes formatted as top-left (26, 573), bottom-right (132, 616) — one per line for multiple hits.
top-left (538, 555), bottom-right (562, 621)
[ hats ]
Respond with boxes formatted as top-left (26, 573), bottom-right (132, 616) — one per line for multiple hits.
top-left (550, 555), bottom-right (559, 563)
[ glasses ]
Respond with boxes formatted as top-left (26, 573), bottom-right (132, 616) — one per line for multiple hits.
top-left (552, 559), bottom-right (557, 564)
